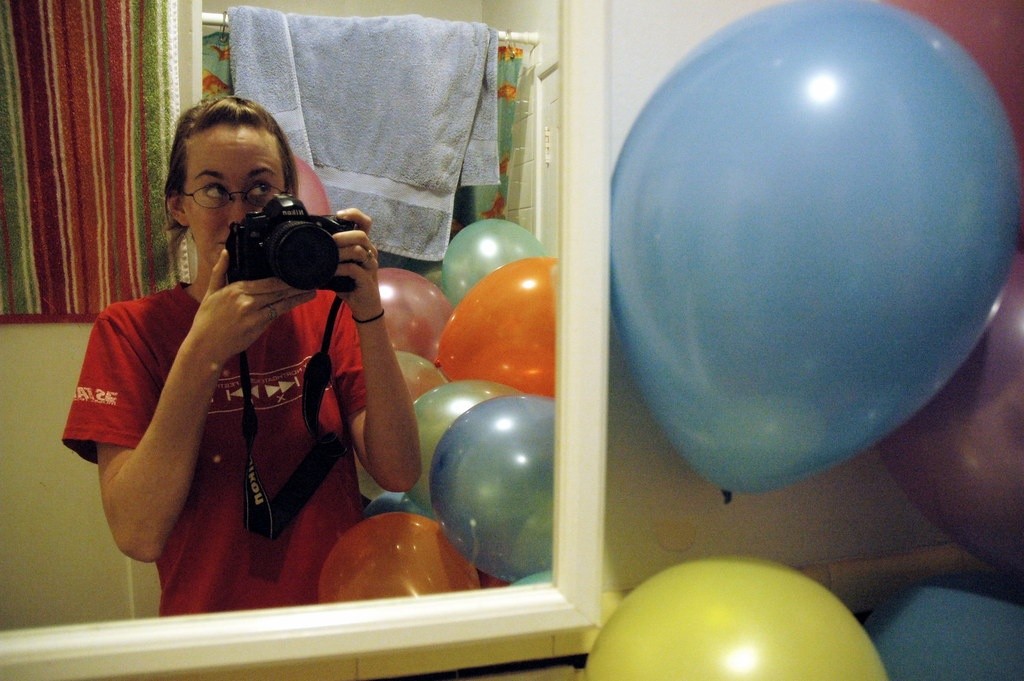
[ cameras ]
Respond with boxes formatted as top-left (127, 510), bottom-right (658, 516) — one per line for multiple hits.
top-left (225, 192), bottom-right (361, 293)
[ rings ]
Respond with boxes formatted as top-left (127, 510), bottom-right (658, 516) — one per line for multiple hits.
top-left (268, 304), bottom-right (278, 323)
top-left (362, 250), bottom-right (373, 266)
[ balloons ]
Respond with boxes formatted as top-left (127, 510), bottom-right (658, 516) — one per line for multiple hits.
top-left (582, 0), bottom-right (1024, 681)
top-left (318, 214), bottom-right (556, 602)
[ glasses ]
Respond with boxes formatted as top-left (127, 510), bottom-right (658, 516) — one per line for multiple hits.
top-left (177, 182), bottom-right (290, 210)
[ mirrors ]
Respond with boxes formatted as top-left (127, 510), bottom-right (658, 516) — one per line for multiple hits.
top-left (0, 0), bottom-right (613, 681)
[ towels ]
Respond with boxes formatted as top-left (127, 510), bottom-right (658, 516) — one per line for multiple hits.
top-left (225, 5), bottom-right (504, 262)
top-left (0, 0), bottom-right (191, 324)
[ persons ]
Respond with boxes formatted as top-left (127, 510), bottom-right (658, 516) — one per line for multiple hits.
top-left (62, 98), bottom-right (422, 617)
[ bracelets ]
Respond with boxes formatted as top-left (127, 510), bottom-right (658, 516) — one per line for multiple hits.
top-left (353, 311), bottom-right (385, 327)
